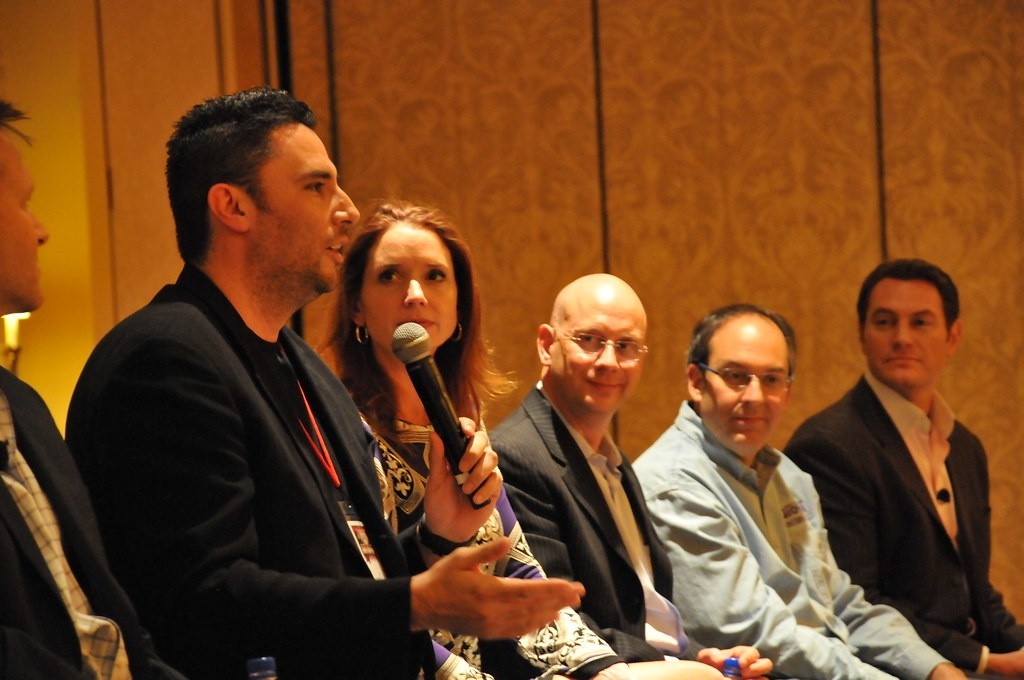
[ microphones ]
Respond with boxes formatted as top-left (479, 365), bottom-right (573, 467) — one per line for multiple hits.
top-left (391, 322), bottom-right (491, 510)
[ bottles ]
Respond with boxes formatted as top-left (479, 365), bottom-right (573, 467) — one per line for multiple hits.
top-left (248, 656), bottom-right (278, 680)
top-left (720, 658), bottom-right (741, 680)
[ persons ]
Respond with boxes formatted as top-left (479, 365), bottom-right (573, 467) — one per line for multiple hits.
top-left (783, 257), bottom-right (1024, 679)
top-left (330, 201), bottom-right (729, 680)
top-left (632, 303), bottom-right (971, 679)
top-left (486, 274), bottom-right (774, 679)
top-left (65, 90), bottom-right (585, 680)
top-left (0, 97), bottom-right (192, 680)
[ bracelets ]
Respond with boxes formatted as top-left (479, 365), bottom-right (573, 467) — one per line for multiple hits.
top-left (417, 523), bottom-right (479, 558)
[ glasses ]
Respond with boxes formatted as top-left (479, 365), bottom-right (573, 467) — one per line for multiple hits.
top-left (699, 363), bottom-right (792, 393)
top-left (556, 328), bottom-right (648, 361)
